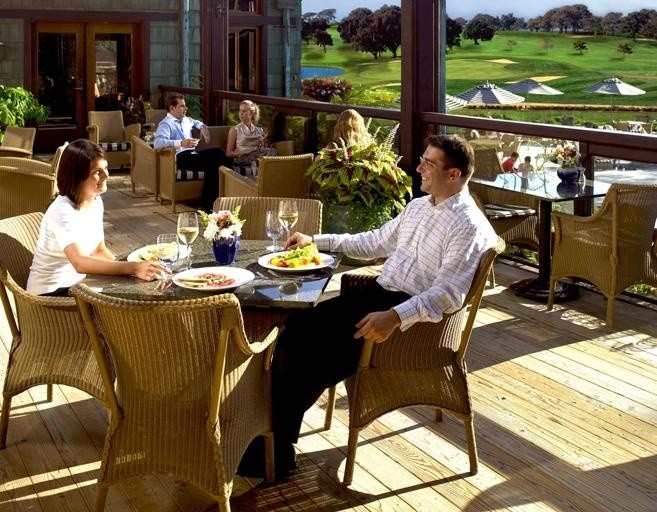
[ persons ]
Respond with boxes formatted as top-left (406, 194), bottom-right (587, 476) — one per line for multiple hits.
top-left (501, 150), bottom-right (517, 173)
top-left (26, 138), bottom-right (171, 298)
top-left (238, 134), bottom-right (497, 478)
top-left (153, 92), bottom-right (231, 211)
top-left (226, 99), bottom-right (276, 170)
top-left (317, 109), bottom-right (375, 151)
top-left (519, 156), bottom-right (533, 172)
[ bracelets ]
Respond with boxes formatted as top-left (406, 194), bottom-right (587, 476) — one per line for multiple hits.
top-left (200, 124), bottom-right (207, 128)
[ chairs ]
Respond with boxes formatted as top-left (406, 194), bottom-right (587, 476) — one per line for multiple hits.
top-left (2, 126), bottom-right (39, 159)
top-left (2, 210), bottom-right (115, 451)
top-left (212, 195), bottom-right (325, 242)
top-left (547, 182), bottom-right (657, 330)
top-left (68, 279), bottom-right (280, 511)
top-left (1, 140), bottom-right (71, 217)
top-left (85, 110), bottom-right (142, 173)
top-left (465, 144), bottom-right (545, 290)
top-left (128, 105), bottom-right (317, 213)
top-left (322, 241), bottom-right (499, 486)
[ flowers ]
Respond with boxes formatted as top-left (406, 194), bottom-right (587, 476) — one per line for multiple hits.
top-left (197, 206), bottom-right (248, 242)
top-left (547, 141), bottom-right (584, 166)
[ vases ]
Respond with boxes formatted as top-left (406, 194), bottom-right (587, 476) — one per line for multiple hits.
top-left (212, 235), bottom-right (240, 265)
top-left (558, 167), bottom-right (584, 183)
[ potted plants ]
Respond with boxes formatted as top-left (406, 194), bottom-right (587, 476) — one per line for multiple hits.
top-left (308, 125), bottom-right (414, 265)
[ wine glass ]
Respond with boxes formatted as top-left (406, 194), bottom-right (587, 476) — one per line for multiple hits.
top-left (176, 211), bottom-right (200, 270)
top-left (276, 199), bottom-right (298, 252)
top-left (155, 232), bottom-right (179, 283)
top-left (263, 210), bottom-right (283, 253)
top-left (190, 128), bottom-right (203, 155)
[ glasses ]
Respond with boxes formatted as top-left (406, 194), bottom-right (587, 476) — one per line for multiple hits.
top-left (419, 156), bottom-right (435, 170)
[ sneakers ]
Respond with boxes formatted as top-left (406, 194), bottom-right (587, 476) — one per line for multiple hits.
top-left (236, 445), bottom-right (299, 484)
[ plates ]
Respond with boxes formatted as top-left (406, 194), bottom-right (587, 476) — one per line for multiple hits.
top-left (176, 266), bottom-right (254, 292)
top-left (258, 250), bottom-right (334, 272)
top-left (126, 242), bottom-right (193, 265)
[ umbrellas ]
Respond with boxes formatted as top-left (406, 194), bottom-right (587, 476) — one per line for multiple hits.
top-left (394, 86), bottom-right (467, 113)
top-left (582, 75), bottom-right (647, 124)
top-left (504, 78), bottom-right (565, 121)
top-left (456, 79), bottom-right (526, 117)
